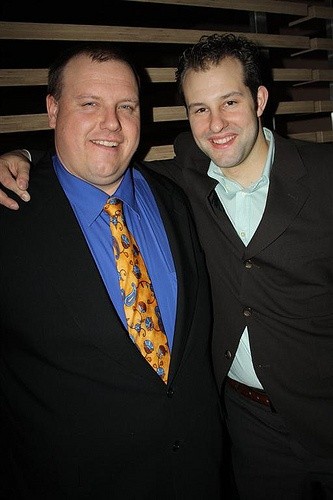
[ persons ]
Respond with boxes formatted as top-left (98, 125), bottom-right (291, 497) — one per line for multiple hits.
top-left (0, 30), bottom-right (333, 500)
top-left (0, 43), bottom-right (214, 500)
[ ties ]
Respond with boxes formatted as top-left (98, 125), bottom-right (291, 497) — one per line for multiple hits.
top-left (104, 203), bottom-right (171, 384)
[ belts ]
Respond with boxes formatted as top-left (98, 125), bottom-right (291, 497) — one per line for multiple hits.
top-left (227, 378), bottom-right (270, 406)
